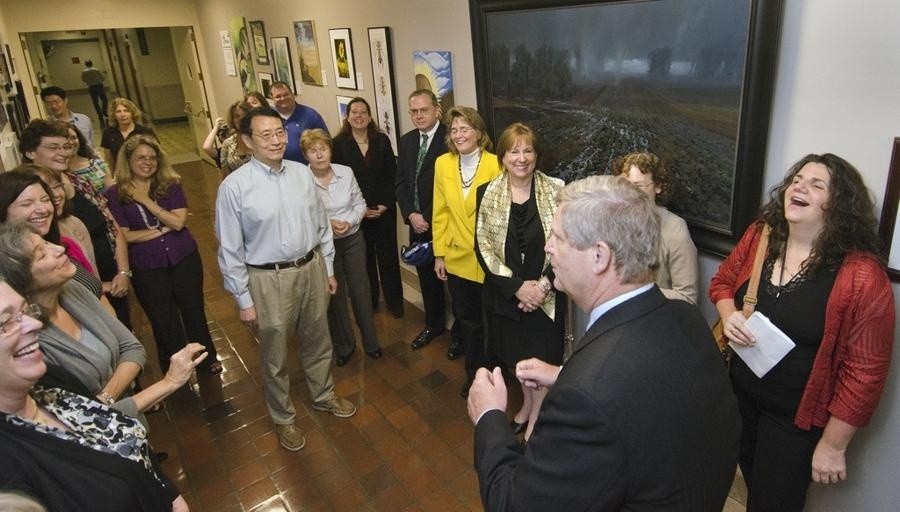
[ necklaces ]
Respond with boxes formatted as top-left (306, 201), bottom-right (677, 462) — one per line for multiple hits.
top-left (457, 151), bottom-right (482, 188)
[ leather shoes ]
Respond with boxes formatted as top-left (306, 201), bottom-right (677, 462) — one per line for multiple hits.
top-left (312, 396), bottom-right (356, 418)
top-left (335, 300), bottom-right (530, 448)
top-left (276, 423), bottom-right (306, 451)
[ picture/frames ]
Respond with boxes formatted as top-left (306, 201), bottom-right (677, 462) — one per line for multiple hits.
top-left (468, 0), bottom-right (785, 262)
top-left (875, 137), bottom-right (900, 283)
top-left (367, 26), bottom-right (401, 158)
top-left (270, 36), bottom-right (298, 96)
top-left (258, 72), bottom-right (275, 100)
top-left (249, 20), bottom-right (270, 65)
top-left (336, 95), bottom-right (355, 129)
top-left (328, 27), bottom-right (358, 90)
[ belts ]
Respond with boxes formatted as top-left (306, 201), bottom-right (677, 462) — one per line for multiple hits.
top-left (246, 249), bottom-right (314, 270)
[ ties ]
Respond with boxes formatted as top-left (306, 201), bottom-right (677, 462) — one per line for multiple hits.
top-left (413, 135), bottom-right (429, 213)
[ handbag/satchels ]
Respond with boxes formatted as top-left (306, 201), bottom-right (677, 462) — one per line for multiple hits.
top-left (710, 316), bottom-right (735, 363)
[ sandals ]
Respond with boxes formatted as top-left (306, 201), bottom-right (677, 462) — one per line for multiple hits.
top-left (206, 359), bottom-right (222, 374)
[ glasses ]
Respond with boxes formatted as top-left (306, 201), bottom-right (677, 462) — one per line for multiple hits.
top-left (251, 130), bottom-right (286, 140)
top-left (0, 303), bottom-right (42, 337)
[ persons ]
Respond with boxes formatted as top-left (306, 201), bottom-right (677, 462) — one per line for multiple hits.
top-left (613, 152), bottom-right (700, 308)
top-left (1, 86), bottom-right (222, 512)
top-left (704, 152), bottom-right (897, 512)
top-left (473, 122), bottom-right (568, 444)
top-left (466, 174), bottom-right (742, 512)
top-left (430, 103), bottom-right (504, 398)
top-left (80, 58), bottom-right (109, 120)
top-left (203, 83), bottom-right (464, 451)
